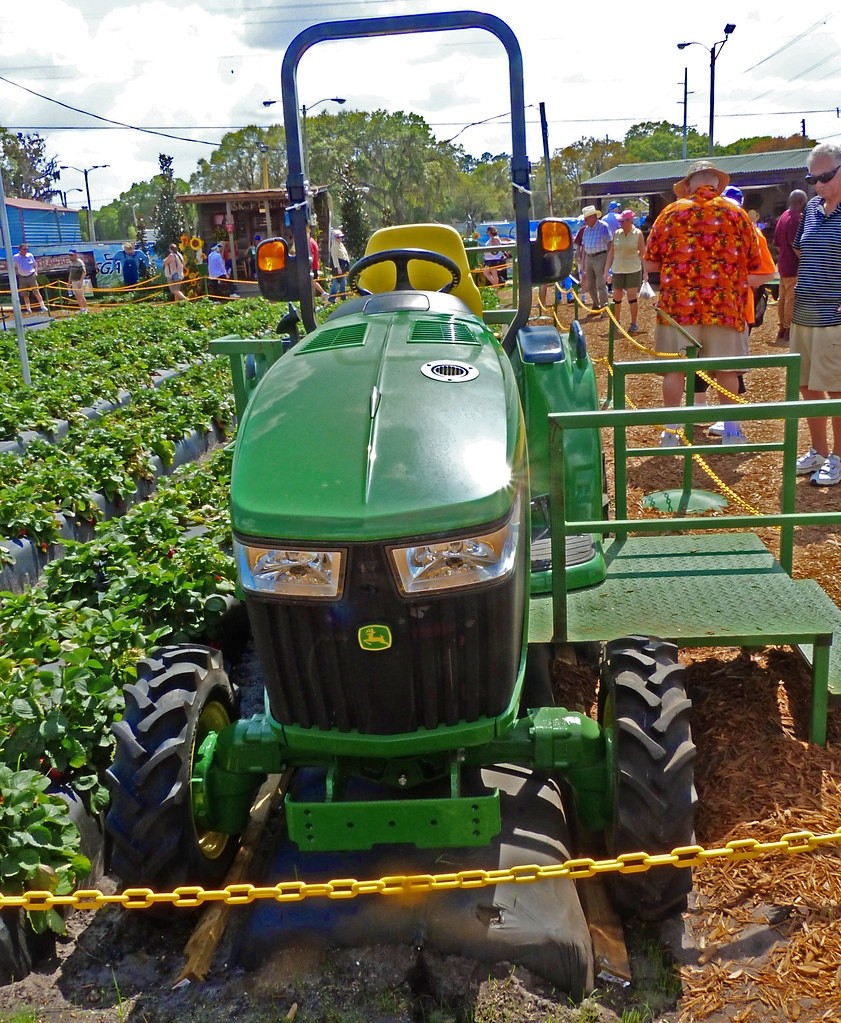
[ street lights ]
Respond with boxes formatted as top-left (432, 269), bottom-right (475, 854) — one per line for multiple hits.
top-left (263, 96), bottom-right (345, 184)
top-left (676, 23), bottom-right (736, 156)
top-left (133, 203), bottom-right (139, 227)
top-left (60, 165), bottom-right (111, 240)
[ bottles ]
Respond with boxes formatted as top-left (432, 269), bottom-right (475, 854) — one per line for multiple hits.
top-left (68, 290), bottom-right (73, 296)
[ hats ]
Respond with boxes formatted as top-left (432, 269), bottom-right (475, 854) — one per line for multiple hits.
top-left (618, 210), bottom-right (635, 220)
top-left (67, 249), bottom-right (77, 255)
top-left (210, 242), bottom-right (220, 250)
top-left (578, 205), bottom-right (602, 220)
top-left (726, 189), bottom-right (743, 197)
top-left (747, 210), bottom-right (760, 222)
top-left (608, 201), bottom-right (621, 210)
top-left (334, 230), bottom-right (344, 238)
top-left (673, 161), bottom-right (729, 197)
top-left (255, 236), bottom-right (260, 240)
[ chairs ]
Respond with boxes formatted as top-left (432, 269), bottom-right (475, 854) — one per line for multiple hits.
top-left (237, 255), bottom-right (251, 279)
top-left (353, 222), bottom-right (483, 318)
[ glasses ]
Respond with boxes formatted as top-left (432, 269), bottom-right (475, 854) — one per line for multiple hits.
top-left (806, 165), bottom-right (840, 185)
top-left (618, 220), bottom-right (624, 223)
top-left (337, 237), bottom-right (343, 239)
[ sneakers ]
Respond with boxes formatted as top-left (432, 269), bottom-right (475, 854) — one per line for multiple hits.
top-left (659, 429), bottom-right (679, 447)
top-left (708, 420), bottom-right (724, 435)
top-left (722, 432), bottom-right (748, 444)
top-left (810, 452), bottom-right (841, 487)
top-left (795, 448), bottom-right (833, 476)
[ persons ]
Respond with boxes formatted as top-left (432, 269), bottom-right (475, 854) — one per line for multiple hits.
top-left (307, 227), bottom-right (320, 279)
top-left (329, 230), bottom-right (350, 303)
top-left (537, 201), bottom-right (647, 334)
top-left (773, 189), bottom-right (808, 341)
top-left (482, 226), bottom-right (503, 295)
top-left (641, 160), bottom-right (776, 446)
top-left (789, 144), bottom-right (841, 487)
top-left (288, 241), bottom-right (331, 305)
top-left (207, 242), bottom-right (241, 297)
top-left (67, 249), bottom-right (89, 314)
top-left (163, 244), bottom-right (186, 302)
top-left (244, 235), bottom-right (261, 280)
top-left (12, 244), bottom-right (48, 314)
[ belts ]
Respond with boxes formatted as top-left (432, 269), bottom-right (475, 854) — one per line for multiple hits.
top-left (19, 272), bottom-right (34, 278)
top-left (585, 251), bottom-right (607, 257)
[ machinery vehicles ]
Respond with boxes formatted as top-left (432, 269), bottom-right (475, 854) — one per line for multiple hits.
top-left (100, 8), bottom-right (841, 941)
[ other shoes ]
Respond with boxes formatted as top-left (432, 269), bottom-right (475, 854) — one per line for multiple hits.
top-left (557, 291), bottom-right (615, 318)
top-left (230, 293), bottom-right (240, 298)
top-left (776, 322), bottom-right (789, 341)
top-left (628, 322), bottom-right (640, 332)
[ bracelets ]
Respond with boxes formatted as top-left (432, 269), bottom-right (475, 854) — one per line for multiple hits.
top-left (79, 282), bottom-right (82, 284)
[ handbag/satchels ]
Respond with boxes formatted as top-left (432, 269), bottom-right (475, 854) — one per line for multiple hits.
top-left (639, 279), bottom-right (656, 300)
top-left (331, 257), bottom-right (349, 269)
top-left (171, 273), bottom-right (182, 282)
